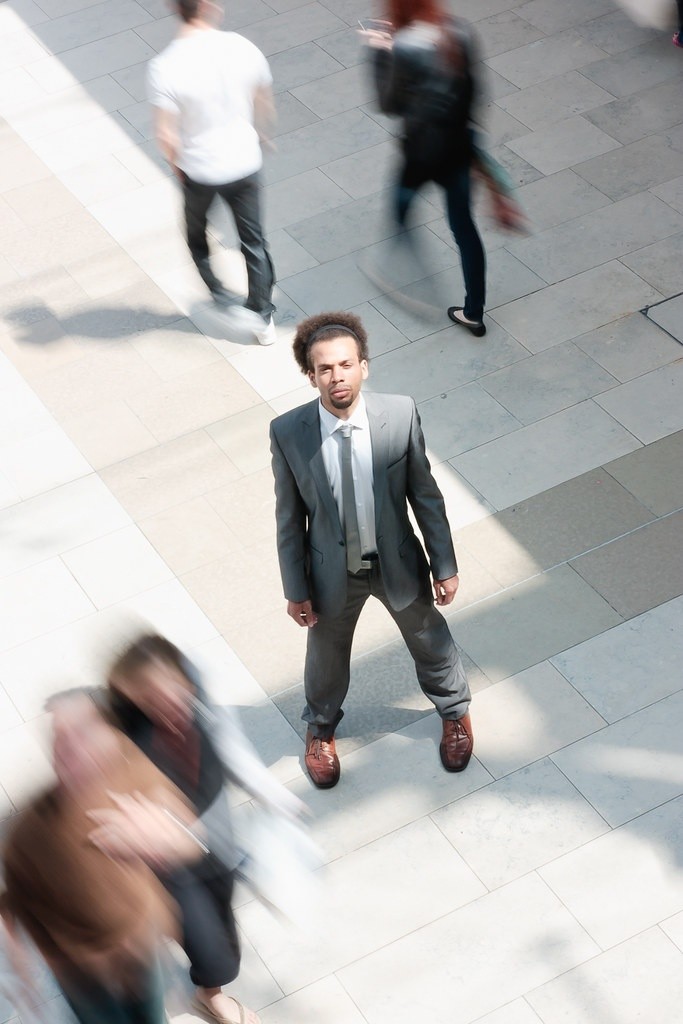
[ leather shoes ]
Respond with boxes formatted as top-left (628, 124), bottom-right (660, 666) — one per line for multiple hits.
top-left (440, 706), bottom-right (474, 773)
top-left (305, 726), bottom-right (340, 788)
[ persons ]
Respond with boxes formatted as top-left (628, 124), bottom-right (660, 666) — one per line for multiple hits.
top-left (269, 313), bottom-right (474, 791)
top-left (148, 1), bottom-right (276, 346)
top-left (362, 1), bottom-right (488, 338)
top-left (0, 636), bottom-right (261, 1023)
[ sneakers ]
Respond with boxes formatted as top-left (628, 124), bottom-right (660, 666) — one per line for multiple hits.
top-left (252, 315), bottom-right (277, 346)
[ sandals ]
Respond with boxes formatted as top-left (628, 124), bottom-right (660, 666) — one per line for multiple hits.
top-left (448, 306), bottom-right (487, 337)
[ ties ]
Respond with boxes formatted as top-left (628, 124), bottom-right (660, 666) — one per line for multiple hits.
top-left (336, 426), bottom-right (362, 575)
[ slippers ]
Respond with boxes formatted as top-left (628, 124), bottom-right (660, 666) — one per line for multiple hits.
top-left (190, 995), bottom-right (264, 1024)
